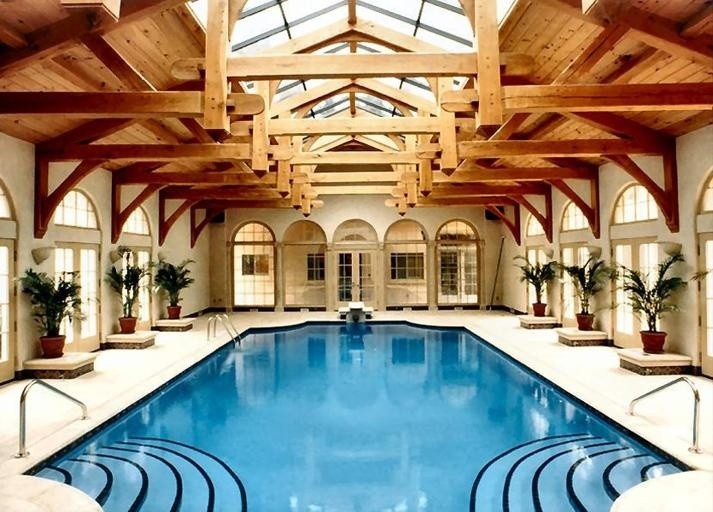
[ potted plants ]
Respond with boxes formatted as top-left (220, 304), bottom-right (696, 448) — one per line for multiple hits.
top-left (152, 253), bottom-right (196, 319)
top-left (614, 248), bottom-right (712, 353)
top-left (15, 263), bottom-right (84, 358)
top-left (512, 253), bottom-right (557, 316)
top-left (106, 259), bottom-right (161, 334)
top-left (553, 254), bottom-right (615, 330)
